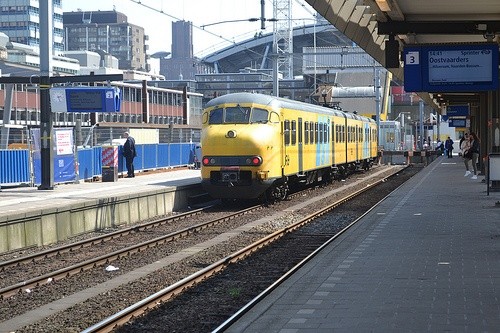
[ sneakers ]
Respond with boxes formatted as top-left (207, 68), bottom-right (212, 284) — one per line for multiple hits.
top-left (463, 171), bottom-right (471, 177)
top-left (470, 175), bottom-right (477, 179)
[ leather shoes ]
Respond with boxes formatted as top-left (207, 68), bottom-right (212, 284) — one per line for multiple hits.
top-left (127, 174), bottom-right (135, 177)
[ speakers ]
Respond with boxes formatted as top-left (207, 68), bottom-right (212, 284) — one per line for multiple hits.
top-left (385, 40), bottom-right (399, 68)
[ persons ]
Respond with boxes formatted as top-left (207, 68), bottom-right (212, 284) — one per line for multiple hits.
top-left (464, 134), bottom-right (480, 179)
top-left (122, 131), bottom-right (136, 178)
top-left (459, 133), bottom-right (474, 172)
top-left (445, 137), bottom-right (454, 158)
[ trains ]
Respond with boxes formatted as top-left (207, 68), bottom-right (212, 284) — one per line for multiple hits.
top-left (198, 90), bottom-right (378, 203)
